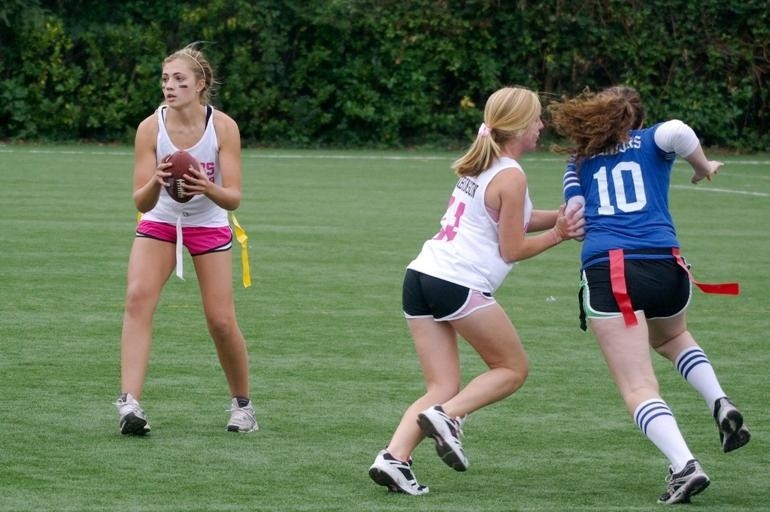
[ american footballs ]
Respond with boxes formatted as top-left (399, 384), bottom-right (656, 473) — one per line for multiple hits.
top-left (163, 150), bottom-right (200, 203)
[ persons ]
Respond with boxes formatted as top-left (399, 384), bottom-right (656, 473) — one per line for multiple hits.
top-left (114, 40), bottom-right (260, 434)
top-left (547, 84), bottom-right (752, 505)
top-left (368, 86), bottom-right (586, 498)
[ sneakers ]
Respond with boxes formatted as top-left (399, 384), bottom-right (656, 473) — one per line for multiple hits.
top-left (116, 392), bottom-right (151, 436)
top-left (369, 448), bottom-right (429, 496)
top-left (226, 397), bottom-right (259, 434)
top-left (417, 405), bottom-right (469, 472)
top-left (656, 459), bottom-right (710, 504)
top-left (714, 397), bottom-right (751, 453)
